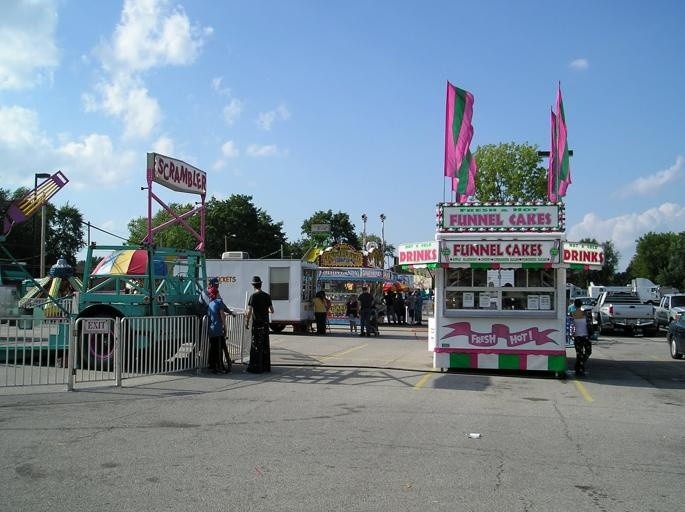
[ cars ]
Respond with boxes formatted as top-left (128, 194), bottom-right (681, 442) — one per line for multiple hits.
top-left (575, 296), bottom-right (598, 311)
top-left (666, 311), bottom-right (685, 359)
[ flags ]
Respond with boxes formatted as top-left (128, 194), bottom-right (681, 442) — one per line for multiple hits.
top-left (444, 75), bottom-right (572, 207)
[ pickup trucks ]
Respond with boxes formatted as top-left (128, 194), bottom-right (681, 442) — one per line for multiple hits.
top-left (653, 293), bottom-right (685, 329)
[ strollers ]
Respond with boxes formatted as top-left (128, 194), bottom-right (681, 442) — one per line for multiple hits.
top-left (363, 308), bottom-right (380, 337)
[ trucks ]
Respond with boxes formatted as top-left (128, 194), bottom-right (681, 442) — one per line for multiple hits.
top-left (591, 290), bottom-right (654, 336)
top-left (589, 277), bottom-right (662, 305)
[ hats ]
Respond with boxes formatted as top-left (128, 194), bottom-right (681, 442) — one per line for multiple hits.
top-left (208, 287), bottom-right (217, 300)
top-left (209, 278), bottom-right (219, 286)
top-left (250, 276), bottom-right (263, 284)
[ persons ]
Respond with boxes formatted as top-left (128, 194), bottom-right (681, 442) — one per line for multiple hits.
top-left (502, 282), bottom-right (514, 309)
top-left (307, 291), bottom-right (327, 333)
top-left (195, 276), bottom-right (236, 375)
top-left (320, 290), bottom-right (331, 332)
top-left (244, 275), bottom-right (275, 375)
top-left (344, 294), bottom-right (359, 333)
top-left (358, 286), bottom-right (373, 335)
top-left (204, 288), bottom-right (241, 376)
top-left (571, 299), bottom-right (593, 376)
top-left (369, 310), bottom-right (380, 334)
top-left (385, 290), bottom-right (425, 326)
